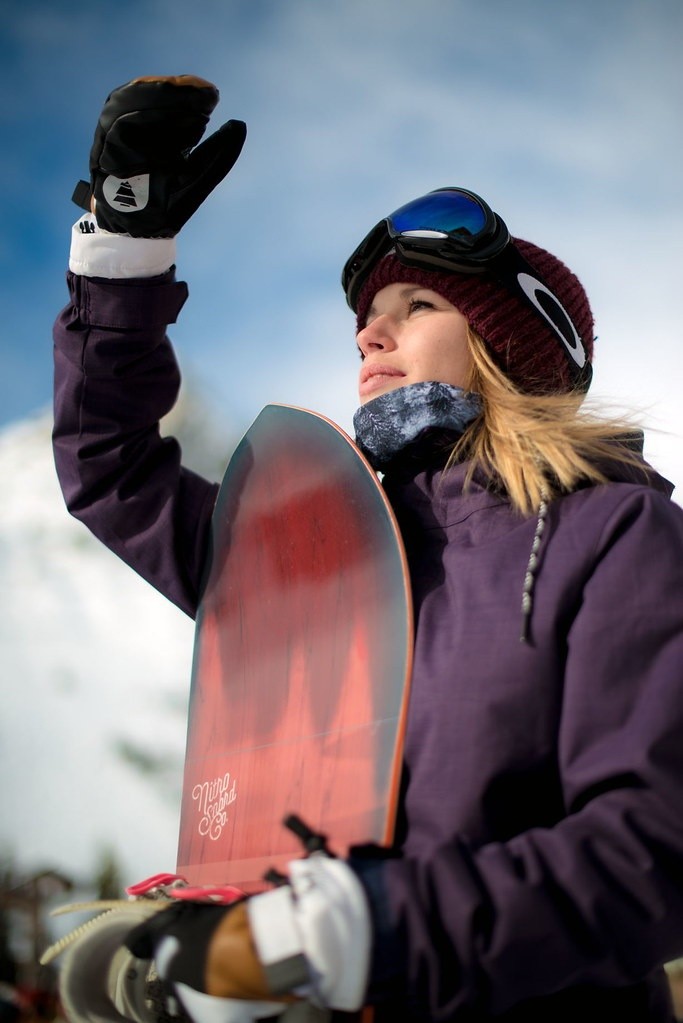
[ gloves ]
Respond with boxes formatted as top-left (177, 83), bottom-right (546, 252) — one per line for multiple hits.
top-left (69, 75), bottom-right (247, 278)
top-left (120, 816), bottom-right (374, 1023)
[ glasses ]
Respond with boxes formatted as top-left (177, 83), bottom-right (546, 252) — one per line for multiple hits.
top-left (341, 186), bottom-right (511, 313)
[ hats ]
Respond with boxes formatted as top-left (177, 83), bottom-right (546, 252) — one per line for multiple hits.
top-left (357, 232), bottom-right (594, 421)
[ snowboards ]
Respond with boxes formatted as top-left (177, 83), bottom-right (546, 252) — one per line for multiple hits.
top-left (174, 396), bottom-right (414, 1022)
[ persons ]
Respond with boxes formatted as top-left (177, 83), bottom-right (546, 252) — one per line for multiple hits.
top-left (39, 75), bottom-right (683, 1023)
